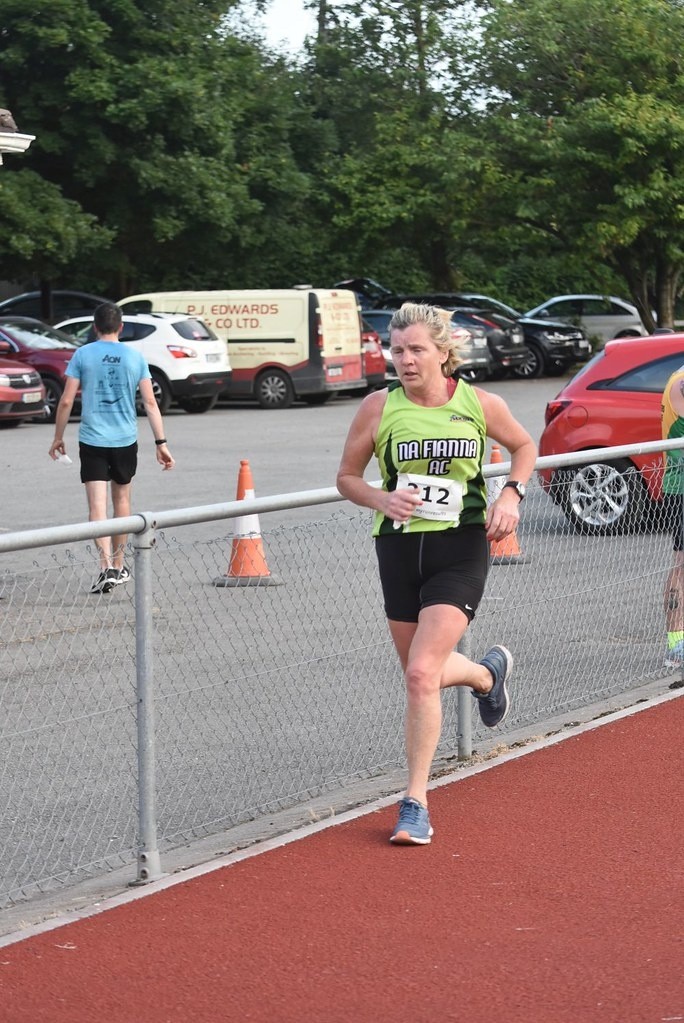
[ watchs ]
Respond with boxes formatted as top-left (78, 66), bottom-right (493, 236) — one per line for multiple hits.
top-left (502, 480), bottom-right (527, 504)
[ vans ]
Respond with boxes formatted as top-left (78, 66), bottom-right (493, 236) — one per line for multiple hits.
top-left (113, 286), bottom-right (371, 409)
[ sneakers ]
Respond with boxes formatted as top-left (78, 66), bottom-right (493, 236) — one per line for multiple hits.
top-left (90, 568), bottom-right (118, 592)
top-left (390, 797), bottom-right (434, 844)
top-left (664, 640), bottom-right (684, 669)
top-left (470, 645), bottom-right (514, 727)
top-left (118, 566), bottom-right (131, 583)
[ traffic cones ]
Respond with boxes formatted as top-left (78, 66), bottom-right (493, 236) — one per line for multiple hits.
top-left (212, 457), bottom-right (287, 586)
top-left (485, 444), bottom-right (536, 564)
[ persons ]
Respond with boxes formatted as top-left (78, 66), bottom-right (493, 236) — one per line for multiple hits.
top-left (336, 301), bottom-right (537, 844)
top-left (48, 302), bottom-right (175, 593)
top-left (660, 364), bottom-right (684, 666)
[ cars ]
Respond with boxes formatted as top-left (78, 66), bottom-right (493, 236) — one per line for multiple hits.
top-left (0, 316), bottom-right (87, 424)
top-left (332, 275), bottom-right (592, 399)
top-left (0, 358), bottom-right (49, 428)
top-left (523, 292), bottom-right (658, 355)
top-left (535, 331), bottom-right (684, 535)
top-left (0, 290), bottom-right (117, 337)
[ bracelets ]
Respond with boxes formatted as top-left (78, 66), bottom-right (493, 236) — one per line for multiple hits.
top-left (155, 438), bottom-right (167, 445)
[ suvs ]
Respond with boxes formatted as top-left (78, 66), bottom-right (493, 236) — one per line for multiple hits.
top-left (23, 310), bottom-right (233, 417)
